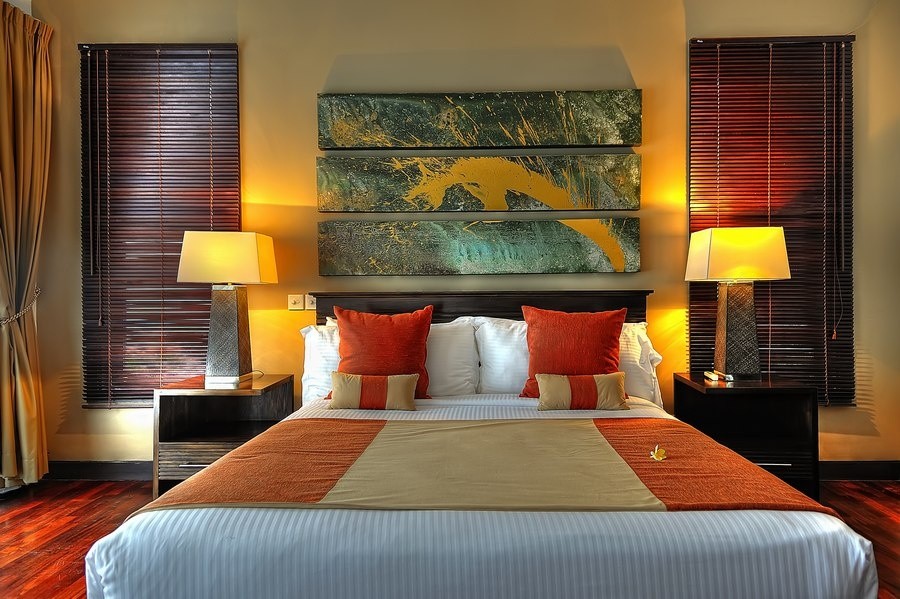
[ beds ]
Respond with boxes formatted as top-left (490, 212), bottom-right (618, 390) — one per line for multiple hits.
top-left (85, 290), bottom-right (879, 599)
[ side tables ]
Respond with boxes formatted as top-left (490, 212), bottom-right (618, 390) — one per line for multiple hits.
top-left (674, 372), bottom-right (818, 502)
top-left (153, 373), bottom-right (294, 500)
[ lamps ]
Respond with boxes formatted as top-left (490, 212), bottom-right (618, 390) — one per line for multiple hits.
top-left (177, 232), bottom-right (279, 383)
top-left (685, 227), bottom-right (792, 380)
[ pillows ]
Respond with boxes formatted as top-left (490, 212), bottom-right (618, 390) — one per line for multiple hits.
top-left (300, 305), bottom-right (664, 411)
